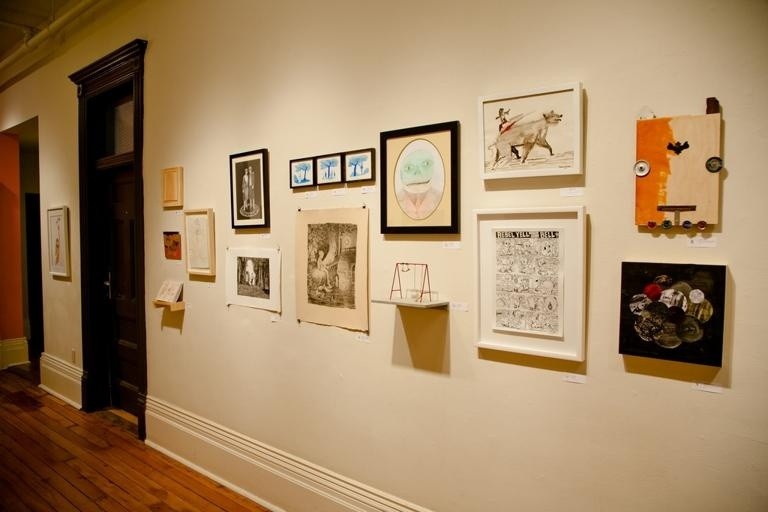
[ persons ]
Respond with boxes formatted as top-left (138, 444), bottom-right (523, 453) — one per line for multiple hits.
top-left (242, 169), bottom-right (249, 211)
top-left (317, 251), bottom-right (333, 289)
top-left (241, 260), bottom-right (255, 286)
top-left (248, 167), bottom-right (255, 211)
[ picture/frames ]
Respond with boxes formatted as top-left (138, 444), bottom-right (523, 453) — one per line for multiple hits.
top-left (229, 148), bottom-right (269, 229)
top-left (477, 80), bottom-right (585, 180)
top-left (182, 207), bottom-right (216, 276)
top-left (289, 147), bottom-right (375, 190)
top-left (46, 205), bottom-right (72, 279)
top-left (380, 118), bottom-right (462, 234)
top-left (475, 206), bottom-right (587, 366)
